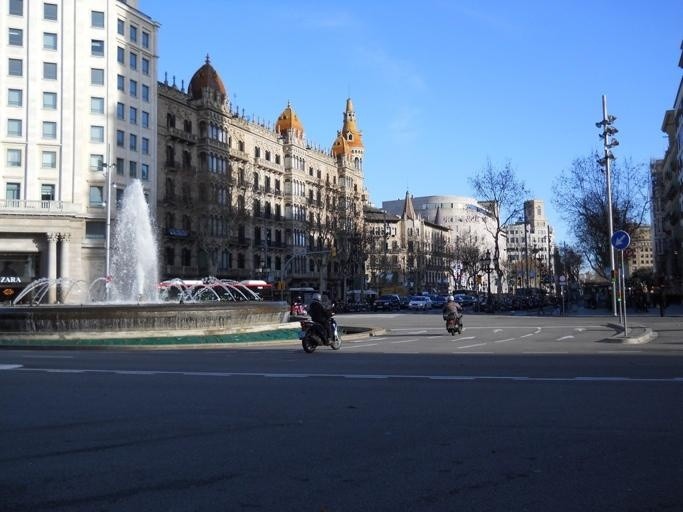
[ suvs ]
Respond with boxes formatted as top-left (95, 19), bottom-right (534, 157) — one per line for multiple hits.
top-left (372, 294), bottom-right (401, 312)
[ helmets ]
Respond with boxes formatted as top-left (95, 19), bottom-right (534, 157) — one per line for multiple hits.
top-left (312, 293), bottom-right (321, 301)
top-left (448, 296), bottom-right (454, 301)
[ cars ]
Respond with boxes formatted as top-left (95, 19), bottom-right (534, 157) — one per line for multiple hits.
top-left (429, 293), bottom-right (478, 307)
top-left (408, 295), bottom-right (432, 311)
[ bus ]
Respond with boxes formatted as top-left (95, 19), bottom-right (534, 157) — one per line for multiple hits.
top-left (159, 279), bottom-right (274, 303)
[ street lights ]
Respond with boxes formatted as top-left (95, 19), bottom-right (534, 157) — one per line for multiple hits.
top-left (101, 143), bottom-right (118, 301)
top-left (481, 250), bottom-right (498, 313)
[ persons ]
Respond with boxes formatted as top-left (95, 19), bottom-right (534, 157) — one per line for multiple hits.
top-left (295, 296), bottom-right (303, 306)
top-left (309, 293), bottom-right (334, 343)
top-left (443, 296), bottom-right (463, 326)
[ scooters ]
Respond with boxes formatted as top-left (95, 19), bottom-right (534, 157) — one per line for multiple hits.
top-left (292, 302), bottom-right (308, 317)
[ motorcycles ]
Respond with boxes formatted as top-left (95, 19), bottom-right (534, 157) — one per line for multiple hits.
top-left (298, 304), bottom-right (342, 353)
top-left (447, 303), bottom-right (463, 336)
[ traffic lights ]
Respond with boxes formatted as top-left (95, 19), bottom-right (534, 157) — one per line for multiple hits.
top-left (611, 270), bottom-right (616, 284)
